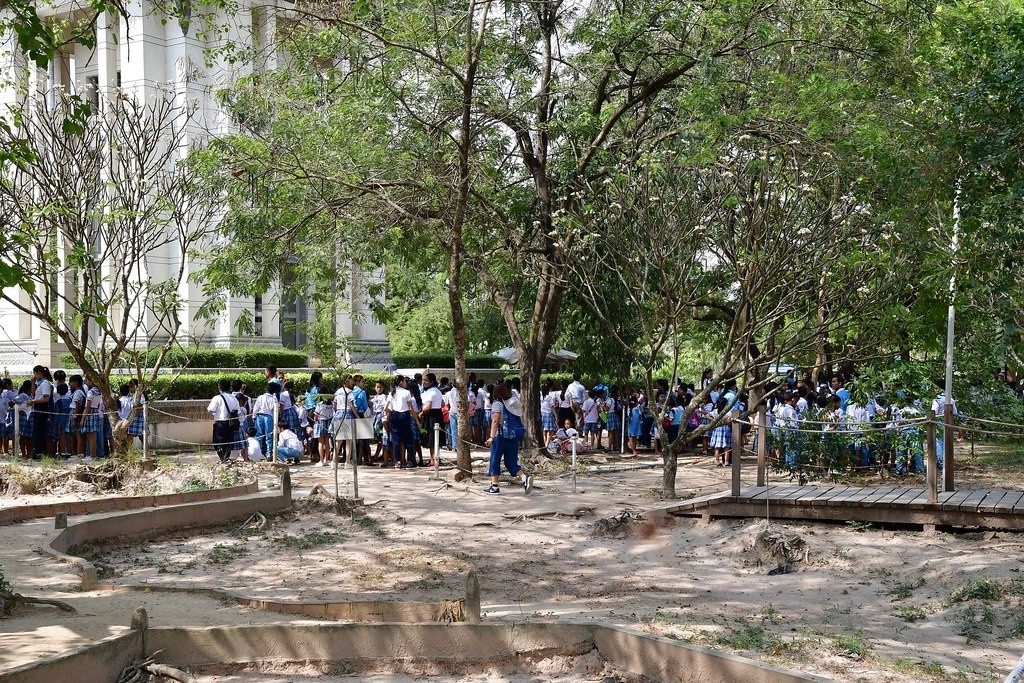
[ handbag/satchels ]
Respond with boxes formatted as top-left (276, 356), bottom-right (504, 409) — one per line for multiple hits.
top-left (577, 417), bottom-right (584, 428)
top-left (227, 410), bottom-right (240, 431)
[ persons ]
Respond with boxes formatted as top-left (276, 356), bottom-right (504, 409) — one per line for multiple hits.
top-left (2, 358), bottom-right (1024, 478)
top-left (481, 383), bottom-right (534, 495)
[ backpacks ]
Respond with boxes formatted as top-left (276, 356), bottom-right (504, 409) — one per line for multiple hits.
top-left (613, 397), bottom-right (622, 415)
top-left (497, 398), bottom-right (526, 441)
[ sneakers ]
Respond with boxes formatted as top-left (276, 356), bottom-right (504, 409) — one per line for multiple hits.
top-left (523, 474), bottom-right (533, 494)
top-left (483, 482), bottom-right (501, 495)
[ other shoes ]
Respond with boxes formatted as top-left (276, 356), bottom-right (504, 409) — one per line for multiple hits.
top-left (324, 461), bottom-right (329, 465)
top-left (287, 461), bottom-right (292, 465)
top-left (725, 464), bottom-right (731, 467)
top-left (68, 457), bottom-right (77, 461)
top-left (596, 445), bottom-right (605, 449)
top-left (590, 447), bottom-right (596, 449)
top-left (634, 451), bottom-right (639, 454)
top-left (81, 456), bottom-right (96, 462)
top-left (716, 463), bottom-right (723, 468)
top-left (655, 450), bottom-right (663, 455)
top-left (294, 459), bottom-right (300, 464)
top-left (316, 461), bottom-right (324, 466)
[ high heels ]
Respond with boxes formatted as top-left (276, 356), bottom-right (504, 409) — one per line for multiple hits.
top-left (331, 461), bottom-right (336, 468)
top-left (345, 462), bottom-right (353, 469)
